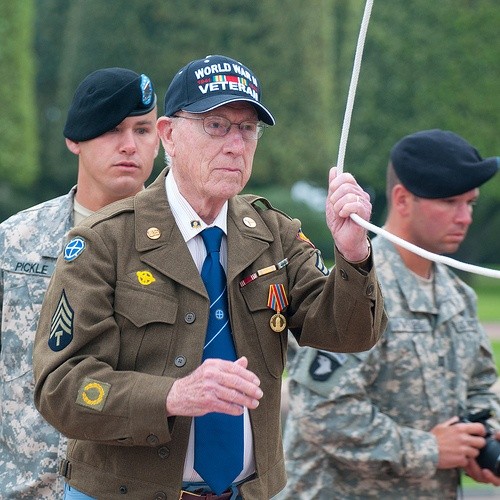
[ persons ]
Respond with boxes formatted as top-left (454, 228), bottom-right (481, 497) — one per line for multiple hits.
top-left (278, 129), bottom-right (500, 500)
top-left (33, 55), bottom-right (387, 500)
top-left (0, 68), bottom-right (161, 500)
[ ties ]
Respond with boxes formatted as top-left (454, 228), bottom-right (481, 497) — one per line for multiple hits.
top-left (194, 224), bottom-right (244, 494)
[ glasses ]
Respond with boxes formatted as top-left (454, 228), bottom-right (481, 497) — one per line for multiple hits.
top-left (170, 114), bottom-right (269, 139)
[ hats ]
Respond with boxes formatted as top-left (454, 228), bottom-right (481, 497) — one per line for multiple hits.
top-left (163, 54), bottom-right (276, 126)
top-left (64, 68), bottom-right (157, 143)
top-left (391, 129), bottom-right (500, 199)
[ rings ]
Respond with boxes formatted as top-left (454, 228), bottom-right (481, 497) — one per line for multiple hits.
top-left (357, 196), bottom-right (359, 201)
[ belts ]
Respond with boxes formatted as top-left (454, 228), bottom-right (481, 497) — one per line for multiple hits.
top-left (180, 485), bottom-right (243, 500)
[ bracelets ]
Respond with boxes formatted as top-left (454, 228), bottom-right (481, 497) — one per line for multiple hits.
top-left (340, 239), bottom-right (370, 264)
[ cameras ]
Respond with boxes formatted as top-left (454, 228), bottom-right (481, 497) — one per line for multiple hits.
top-left (458, 409), bottom-right (500, 478)
